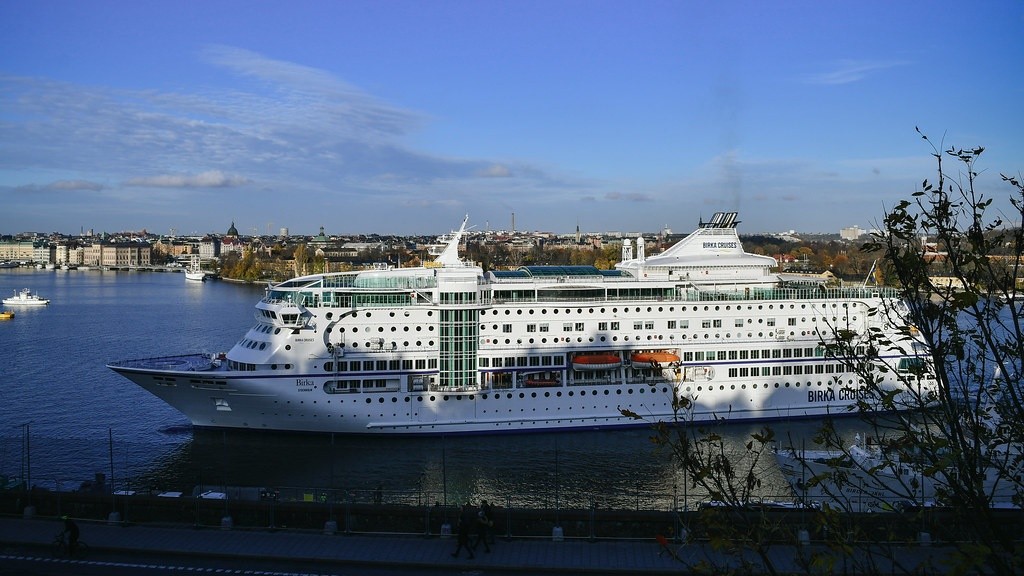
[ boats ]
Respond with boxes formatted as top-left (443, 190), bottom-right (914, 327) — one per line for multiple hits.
top-left (998, 292), bottom-right (1024, 301)
top-left (769, 430), bottom-right (1024, 504)
top-left (1, 290), bottom-right (52, 308)
top-left (182, 249), bottom-right (206, 280)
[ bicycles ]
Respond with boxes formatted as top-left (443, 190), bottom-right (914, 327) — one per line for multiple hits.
top-left (49, 529), bottom-right (88, 560)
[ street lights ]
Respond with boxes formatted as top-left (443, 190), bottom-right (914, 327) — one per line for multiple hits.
top-left (13, 420), bottom-right (35, 495)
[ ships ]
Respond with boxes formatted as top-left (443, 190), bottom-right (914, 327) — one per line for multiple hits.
top-left (106, 209), bottom-right (948, 433)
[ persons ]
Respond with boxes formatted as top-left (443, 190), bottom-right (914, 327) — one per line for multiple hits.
top-left (642, 372), bottom-right (646, 383)
top-left (556, 373), bottom-right (560, 383)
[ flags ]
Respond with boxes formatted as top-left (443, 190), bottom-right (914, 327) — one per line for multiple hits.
top-left (869, 264), bottom-right (878, 286)
